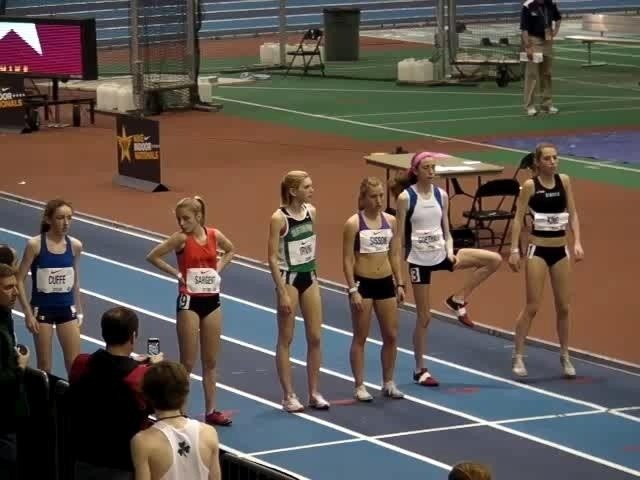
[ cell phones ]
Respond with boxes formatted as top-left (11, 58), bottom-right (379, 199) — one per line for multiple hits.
top-left (147, 338), bottom-right (160, 358)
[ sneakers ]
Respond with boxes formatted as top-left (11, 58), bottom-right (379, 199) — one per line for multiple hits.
top-left (560, 352), bottom-right (576, 376)
top-left (527, 106), bottom-right (536, 116)
top-left (512, 354), bottom-right (527, 377)
top-left (446, 295), bottom-right (474, 327)
top-left (206, 411), bottom-right (232, 425)
top-left (540, 107), bottom-right (559, 114)
top-left (283, 368), bottom-right (439, 411)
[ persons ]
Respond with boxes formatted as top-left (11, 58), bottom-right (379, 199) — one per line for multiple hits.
top-left (15, 198), bottom-right (85, 375)
top-left (131, 360), bottom-right (221, 480)
top-left (268, 170), bottom-right (331, 412)
top-left (389, 150), bottom-right (504, 386)
top-left (448, 462), bottom-right (492, 479)
top-left (519, 0), bottom-right (562, 116)
top-left (342, 176), bottom-right (407, 402)
top-left (1, 241), bottom-right (165, 480)
top-left (146, 197), bottom-right (233, 426)
top-left (508, 142), bottom-right (585, 379)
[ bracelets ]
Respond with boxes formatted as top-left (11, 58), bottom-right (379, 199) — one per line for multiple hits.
top-left (509, 247), bottom-right (519, 254)
top-left (396, 284), bottom-right (405, 290)
top-left (348, 281), bottom-right (360, 294)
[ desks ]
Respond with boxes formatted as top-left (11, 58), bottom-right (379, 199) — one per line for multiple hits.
top-left (363, 151), bottom-right (504, 229)
top-left (565, 35), bottom-right (640, 68)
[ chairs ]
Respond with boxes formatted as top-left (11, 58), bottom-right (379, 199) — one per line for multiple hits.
top-left (488, 152), bottom-right (535, 227)
top-left (284, 28), bottom-right (324, 77)
top-left (463, 179), bottom-right (520, 252)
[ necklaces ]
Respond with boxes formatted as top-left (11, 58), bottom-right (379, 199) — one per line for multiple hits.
top-left (155, 414), bottom-right (188, 422)
